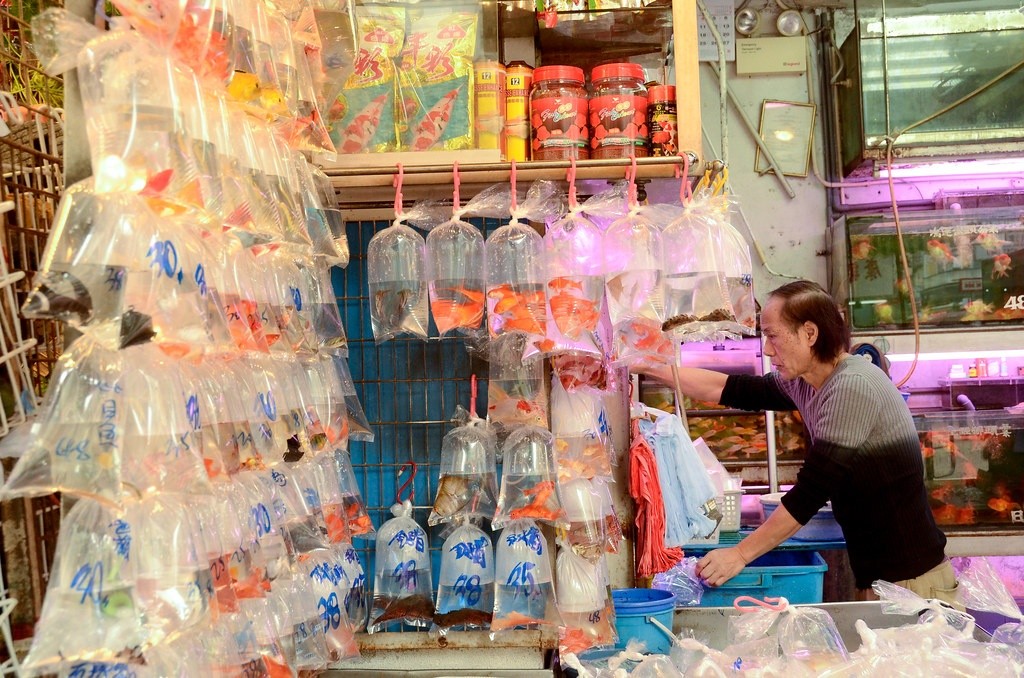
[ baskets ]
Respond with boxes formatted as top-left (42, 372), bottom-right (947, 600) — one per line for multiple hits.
top-left (686, 503), bottom-right (724, 545)
top-left (719, 490), bottom-right (746, 532)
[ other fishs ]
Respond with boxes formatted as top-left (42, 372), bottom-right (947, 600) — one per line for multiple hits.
top-left (368, 434), bottom-right (624, 656)
top-left (22, 0), bottom-right (462, 677)
top-left (369, 267), bottom-right (757, 430)
top-left (918, 421), bottom-right (1022, 525)
top-left (644, 389), bottom-right (807, 462)
top-left (827, 207), bottom-right (1024, 325)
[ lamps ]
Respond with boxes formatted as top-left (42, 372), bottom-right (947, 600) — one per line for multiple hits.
top-left (735, 8), bottom-right (808, 75)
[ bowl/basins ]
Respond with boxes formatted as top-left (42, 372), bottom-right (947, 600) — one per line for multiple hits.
top-left (761, 492), bottom-right (843, 540)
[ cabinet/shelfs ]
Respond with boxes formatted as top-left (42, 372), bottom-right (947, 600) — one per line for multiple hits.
top-left (0, 1), bottom-right (704, 678)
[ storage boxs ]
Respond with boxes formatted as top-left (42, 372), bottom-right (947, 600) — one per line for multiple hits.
top-left (913, 408), bottom-right (1024, 537)
top-left (708, 488), bottom-right (747, 532)
top-left (641, 385), bottom-right (811, 465)
top-left (835, 8), bottom-right (1023, 180)
top-left (828, 204), bottom-right (1023, 332)
top-left (760, 493), bottom-right (844, 541)
top-left (695, 551), bottom-right (828, 607)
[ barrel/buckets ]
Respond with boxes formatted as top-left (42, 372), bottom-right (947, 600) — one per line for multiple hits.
top-left (612, 588), bottom-right (678, 654)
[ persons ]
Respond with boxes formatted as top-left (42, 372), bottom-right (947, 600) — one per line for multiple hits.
top-left (632, 281), bottom-right (961, 604)
